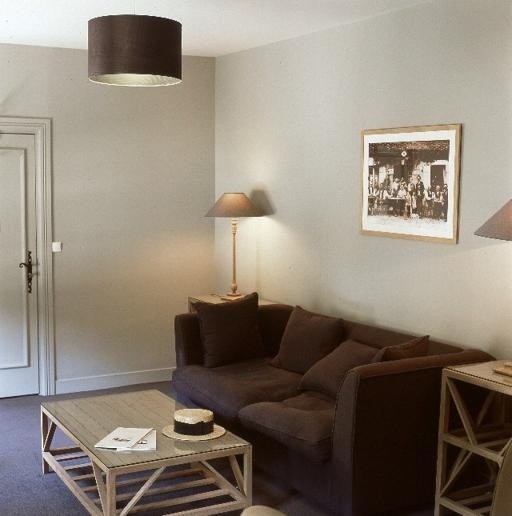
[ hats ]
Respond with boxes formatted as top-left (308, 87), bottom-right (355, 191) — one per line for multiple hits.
top-left (161, 409), bottom-right (226, 441)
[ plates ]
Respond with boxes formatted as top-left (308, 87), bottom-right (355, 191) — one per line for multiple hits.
top-left (162, 424), bottom-right (226, 440)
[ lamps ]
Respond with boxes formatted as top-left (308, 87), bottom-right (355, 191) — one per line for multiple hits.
top-left (202, 192), bottom-right (264, 302)
top-left (87, 0), bottom-right (183, 88)
top-left (472, 198), bottom-right (512, 378)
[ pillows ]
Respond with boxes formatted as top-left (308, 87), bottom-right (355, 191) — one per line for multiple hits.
top-left (371, 334), bottom-right (431, 363)
top-left (296, 337), bottom-right (379, 398)
top-left (194, 292), bottom-right (259, 368)
top-left (268, 305), bottom-right (343, 374)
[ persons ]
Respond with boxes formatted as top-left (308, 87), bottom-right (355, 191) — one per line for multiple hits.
top-left (369, 168), bottom-right (447, 220)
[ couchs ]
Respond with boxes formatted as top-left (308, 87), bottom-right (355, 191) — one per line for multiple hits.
top-left (171, 300), bottom-right (496, 516)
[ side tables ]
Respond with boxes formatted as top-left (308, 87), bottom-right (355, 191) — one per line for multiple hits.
top-left (187, 293), bottom-right (274, 313)
top-left (433, 359), bottom-right (512, 516)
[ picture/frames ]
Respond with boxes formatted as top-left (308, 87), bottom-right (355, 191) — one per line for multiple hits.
top-left (358, 123), bottom-right (462, 246)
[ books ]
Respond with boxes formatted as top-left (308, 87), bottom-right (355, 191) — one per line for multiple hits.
top-left (93, 426), bottom-right (157, 453)
